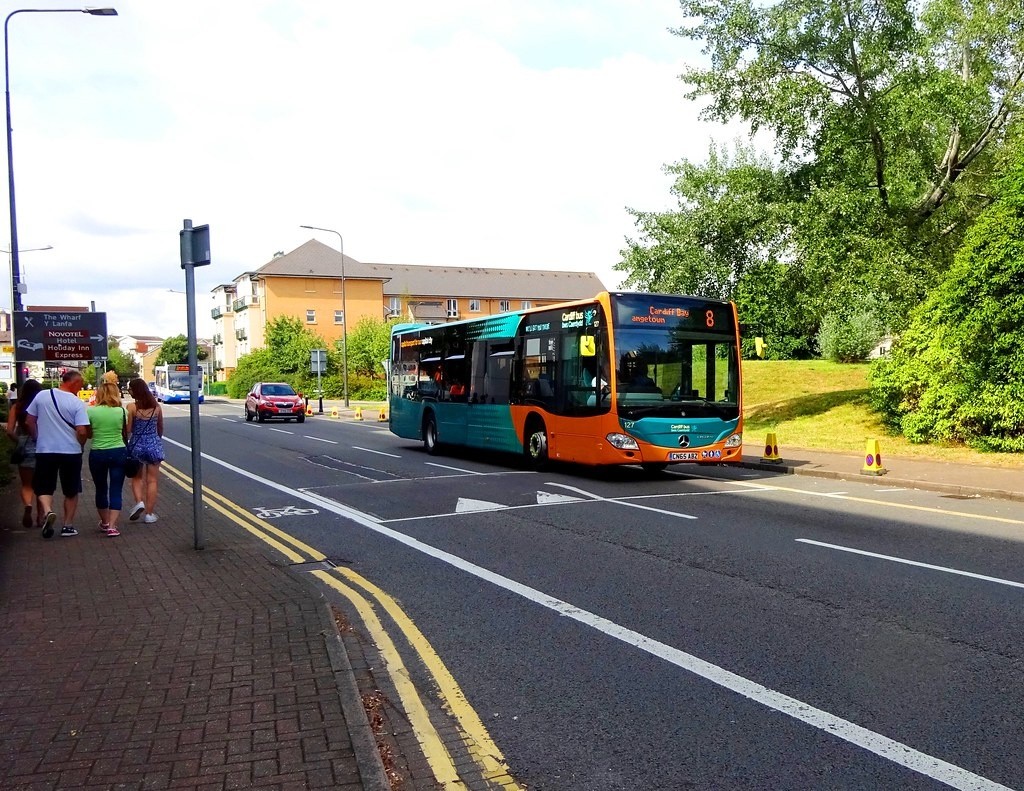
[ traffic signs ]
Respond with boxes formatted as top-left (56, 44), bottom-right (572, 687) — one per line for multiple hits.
top-left (11, 310), bottom-right (109, 362)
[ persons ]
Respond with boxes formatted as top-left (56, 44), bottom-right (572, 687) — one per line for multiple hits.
top-left (126, 379), bottom-right (166, 523)
top-left (6, 380), bottom-right (44, 528)
top-left (7, 383), bottom-right (17, 404)
top-left (86, 383), bottom-right (129, 536)
top-left (591, 362), bottom-right (620, 392)
top-left (25, 371), bottom-right (90, 540)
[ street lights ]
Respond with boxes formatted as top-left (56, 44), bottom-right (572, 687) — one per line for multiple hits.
top-left (3, 7), bottom-right (118, 402)
top-left (300, 224), bottom-right (349, 407)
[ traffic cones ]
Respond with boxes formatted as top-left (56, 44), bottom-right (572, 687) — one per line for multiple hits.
top-left (377, 408), bottom-right (387, 422)
top-left (305, 404), bottom-right (313, 417)
top-left (354, 406), bottom-right (365, 421)
top-left (760, 433), bottom-right (783, 464)
top-left (860, 438), bottom-right (888, 476)
top-left (329, 407), bottom-right (340, 420)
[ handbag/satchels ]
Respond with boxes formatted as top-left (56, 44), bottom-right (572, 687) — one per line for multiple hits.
top-left (126, 459), bottom-right (142, 478)
top-left (10, 447), bottom-right (24, 464)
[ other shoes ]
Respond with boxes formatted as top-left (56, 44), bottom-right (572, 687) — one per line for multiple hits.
top-left (23, 505), bottom-right (33, 528)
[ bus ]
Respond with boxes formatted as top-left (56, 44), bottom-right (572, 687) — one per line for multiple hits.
top-left (155, 361), bottom-right (208, 404)
top-left (385, 291), bottom-right (768, 475)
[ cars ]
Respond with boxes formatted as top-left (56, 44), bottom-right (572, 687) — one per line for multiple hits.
top-left (146, 381), bottom-right (156, 397)
top-left (244, 382), bottom-right (305, 423)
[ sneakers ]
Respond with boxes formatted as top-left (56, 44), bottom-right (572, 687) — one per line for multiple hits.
top-left (99, 520), bottom-right (109, 531)
top-left (129, 503), bottom-right (145, 520)
top-left (146, 513), bottom-right (159, 524)
top-left (41, 513), bottom-right (57, 539)
top-left (107, 526), bottom-right (120, 536)
top-left (61, 526), bottom-right (78, 536)
